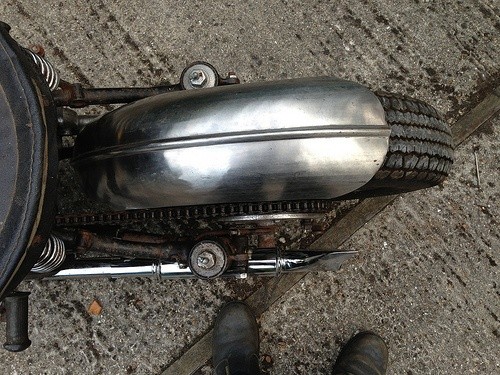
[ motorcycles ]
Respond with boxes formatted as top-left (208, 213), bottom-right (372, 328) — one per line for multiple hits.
top-left (0, 20), bottom-right (458, 355)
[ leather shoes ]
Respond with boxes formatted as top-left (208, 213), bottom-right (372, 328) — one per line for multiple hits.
top-left (333, 332), bottom-right (387, 375)
top-left (211, 302), bottom-right (261, 375)
